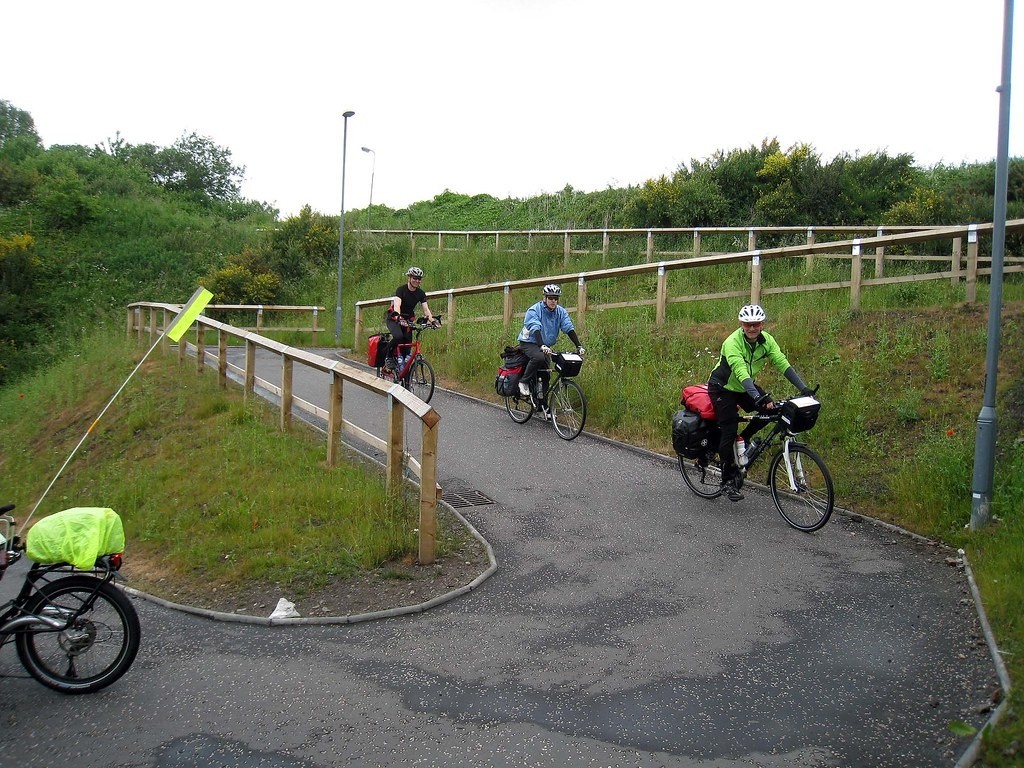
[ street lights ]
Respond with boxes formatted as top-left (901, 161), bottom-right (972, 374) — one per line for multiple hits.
top-left (361, 146), bottom-right (376, 230)
top-left (334, 110), bottom-right (356, 350)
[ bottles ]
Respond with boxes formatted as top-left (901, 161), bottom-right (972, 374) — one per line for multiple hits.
top-left (537, 378), bottom-right (543, 399)
top-left (397, 354), bottom-right (404, 371)
top-left (744, 437), bottom-right (761, 457)
top-left (403, 354), bottom-right (411, 367)
top-left (736, 436), bottom-right (749, 466)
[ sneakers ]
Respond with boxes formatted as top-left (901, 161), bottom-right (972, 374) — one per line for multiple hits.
top-left (518, 382), bottom-right (530, 395)
top-left (539, 412), bottom-right (557, 420)
top-left (720, 482), bottom-right (744, 501)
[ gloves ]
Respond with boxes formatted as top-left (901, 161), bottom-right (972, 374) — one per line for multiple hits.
top-left (578, 345), bottom-right (585, 355)
top-left (540, 344), bottom-right (551, 356)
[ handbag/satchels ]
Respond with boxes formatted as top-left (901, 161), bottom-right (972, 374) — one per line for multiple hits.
top-left (368, 335), bottom-right (386, 366)
top-left (496, 343), bottom-right (526, 396)
top-left (672, 384), bottom-right (741, 460)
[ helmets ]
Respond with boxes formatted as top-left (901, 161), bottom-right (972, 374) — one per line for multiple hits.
top-left (408, 267), bottom-right (423, 277)
top-left (542, 284), bottom-right (562, 296)
top-left (738, 304), bottom-right (765, 322)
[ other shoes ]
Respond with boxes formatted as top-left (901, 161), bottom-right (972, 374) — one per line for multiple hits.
top-left (385, 357), bottom-right (393, 374)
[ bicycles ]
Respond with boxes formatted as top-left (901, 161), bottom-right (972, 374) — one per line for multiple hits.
top-left (675, 383), bottom-right (835, 534)
top-left (495, 345), bottom-right (587, 440)
top-left (368, 312), bottom-right (443, 404)
top-left (0, 503), bottom-right (142, 696)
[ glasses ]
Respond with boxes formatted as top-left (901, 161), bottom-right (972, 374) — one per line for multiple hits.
top-left (413, 276), bottom-right (422, 280)
top-left (547, 296), bottom-right (559, 300)
top-left (742, 323), bottom-right (762, 328)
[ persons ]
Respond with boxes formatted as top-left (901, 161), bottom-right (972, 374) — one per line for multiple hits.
top-left (517, 282), bottom-right (585, 420)
top-left (706, 303), bottom-right (812, 500)
top-left (383, 266), bottom-right (442, 393)
